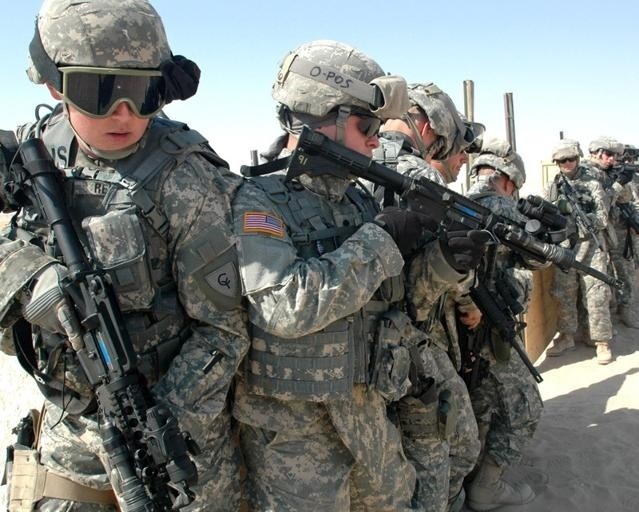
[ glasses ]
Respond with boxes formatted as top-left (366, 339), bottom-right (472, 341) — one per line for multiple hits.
top-left (560, 158), bottom-right (576, 163)
top-left (357, 115), bottom-right (382, 137)
top-left (603, 150), bottom-right (613, 156)
top-left (46, 65), bottom-right (168, 118)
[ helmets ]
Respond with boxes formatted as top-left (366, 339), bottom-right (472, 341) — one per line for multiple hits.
top-left (552, 139), bottom-right (584, 163)
top-left (468, 139), bottom-right (526, 189)
top-left (589, 136), bottom-right (639, 161)
top-left (449, 113), bottom-right (486, 157)
top-left (272, 39), bottom-right (386, 118)
top-left (25, 0), bottom-right (173, 85)
top-left (407, 81), bottom-right (458, 160)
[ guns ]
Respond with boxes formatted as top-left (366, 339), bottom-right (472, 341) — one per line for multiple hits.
top-left (607, 163), bottom-right (639, 237)
top-left (18, 138), bottom-right (197, 512)
top-left (285, 123), bottom-right (627, 293)
top-left (559, 178), bottom-right (606, 250)
top-left (467, 272), bottom-right (545, 384)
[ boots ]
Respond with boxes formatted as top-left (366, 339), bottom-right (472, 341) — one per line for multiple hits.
top-left (467, 462), bottom-right (536, 512)
top-left (594, 341), bottom-right (612, 365)
top-left (546, 333), bottom-right (576, 357)
top-left (617, 303), bottom-right (639, 328)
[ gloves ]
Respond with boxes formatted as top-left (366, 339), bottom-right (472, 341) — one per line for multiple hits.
top-left (446, 228), bottom-right (491, 270)
top-left (372, 204), bottom-right (438, 262)
top-left (16, 263), bottom-right (85, 351)
top-left (558, 199), bottom-right (573, 216)
top-left (616, 167), bottom-right (633, 185)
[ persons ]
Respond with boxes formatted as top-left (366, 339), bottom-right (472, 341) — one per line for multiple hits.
top-left (1, 0), bottom-right (251, 511)
top-left (364, 80), bottom-right (544, 510)
top-left (225, 38), bottom-right (491, 512)
top-left (544, 137), bottom-right (638, 364)
